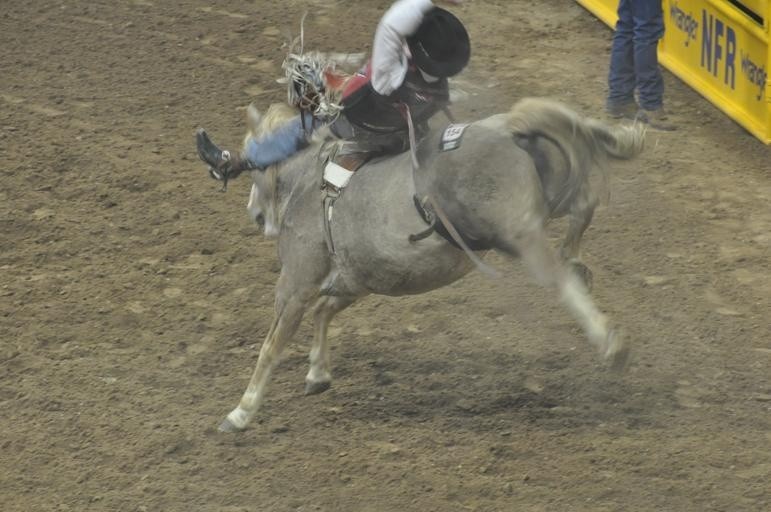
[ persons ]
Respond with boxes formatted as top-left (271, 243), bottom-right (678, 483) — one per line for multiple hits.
top-left (194, 1), bottom-right (471, 180)
top-left (605, 1), bottom-right (677, 130)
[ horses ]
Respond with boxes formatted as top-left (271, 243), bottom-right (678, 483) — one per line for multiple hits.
top-left (215, 96), bottom-right (658, 434)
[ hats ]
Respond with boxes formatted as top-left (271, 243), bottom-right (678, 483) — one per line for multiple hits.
top-left (405, 5), bottom-right (472, 79)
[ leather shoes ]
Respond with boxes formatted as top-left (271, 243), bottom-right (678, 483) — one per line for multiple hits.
top-left (642, 103), bottom-right (677, 132)
top-left (604, 99), bottom-right (643, 120)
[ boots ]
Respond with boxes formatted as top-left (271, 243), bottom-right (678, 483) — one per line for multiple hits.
top-left (192, 125), bottom-right (255, 198)
